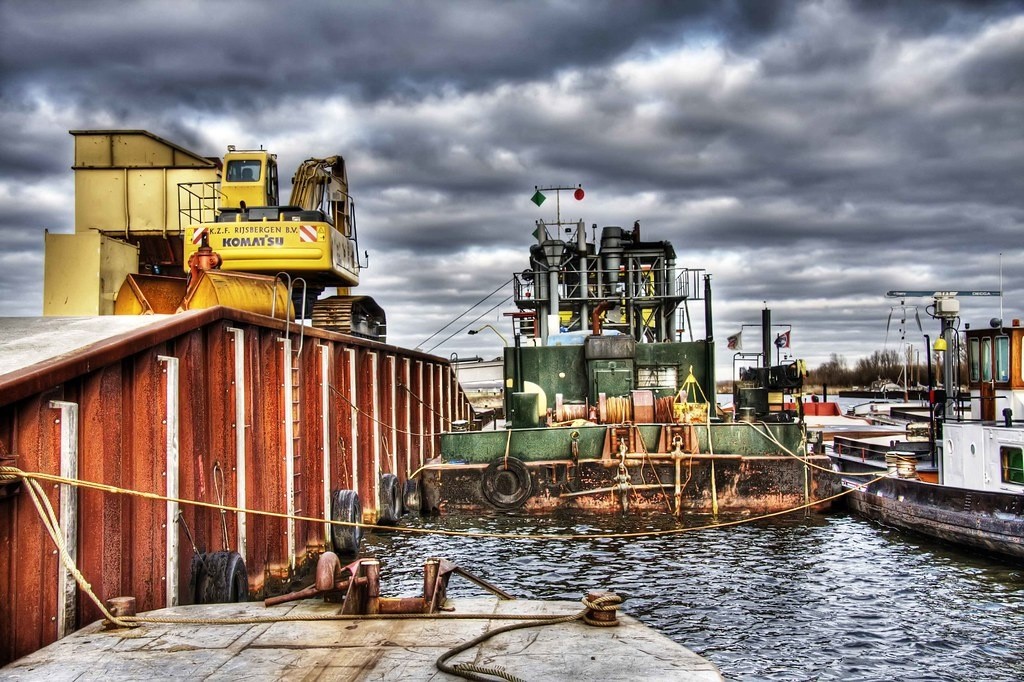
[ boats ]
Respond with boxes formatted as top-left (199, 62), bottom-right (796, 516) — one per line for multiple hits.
top-left (420, 181), bottom-right (843, 513)
top-left (763, 287), bottom-right (1024, 563)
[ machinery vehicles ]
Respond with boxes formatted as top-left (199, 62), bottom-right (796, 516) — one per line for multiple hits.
top-left (181, 143), bottom-right (388, 343)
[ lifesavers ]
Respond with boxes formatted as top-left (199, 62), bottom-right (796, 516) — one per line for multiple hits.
top-left (330, 490), bottom-right (362, 555)
top-left (403, 478), bottom-right (422, 515)
top-left (196, 550), bottom-right (249, 602)
top-left (481, 456), bottom-right (532, 508)
top-left (379, 473), bottom-right (403, 521)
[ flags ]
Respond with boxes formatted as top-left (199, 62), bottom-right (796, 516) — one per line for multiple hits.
top-left (727, 331), bottom-right (743, 350)
top-left (774, 331), bottom-right (790, 348)
